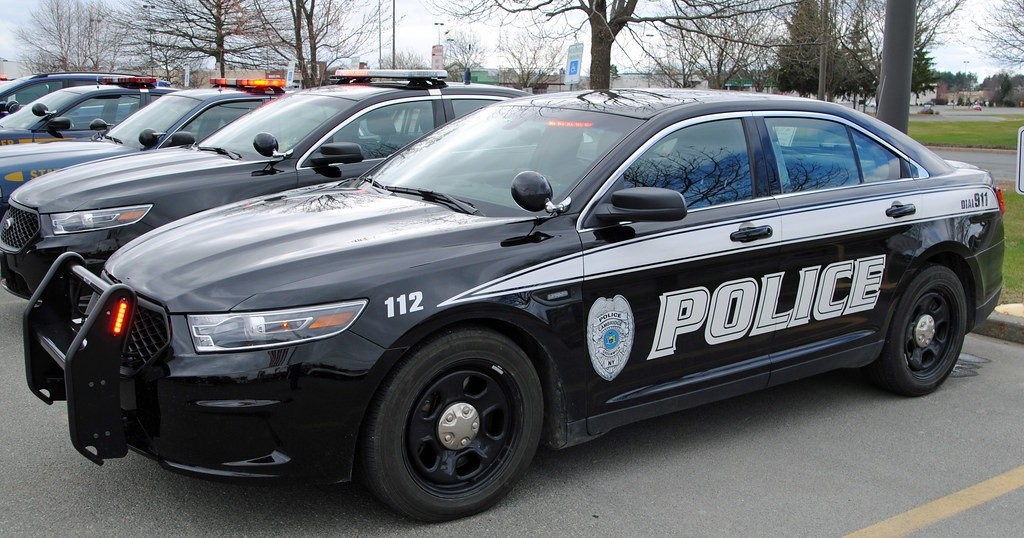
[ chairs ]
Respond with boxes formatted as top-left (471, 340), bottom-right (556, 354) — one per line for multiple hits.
top-left (332, 118), bottom-right (360, 143)
top-left (667, 124), bottom-right (747, 203)
top-left (364, 111), bottom-right (399, 153)
top-left (529, 127), bottom-right (582, 180)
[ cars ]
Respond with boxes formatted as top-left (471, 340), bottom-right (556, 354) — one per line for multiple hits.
top-left (16, 87), bottom-right (1007, 523)
top-left (0, 68), bottom-right (551, 304)
top-left (5, 77), bottom-right (379, 223)
top-left (0, 69), bottom-right (186, 153)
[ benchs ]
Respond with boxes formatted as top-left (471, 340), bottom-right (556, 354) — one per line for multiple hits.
top-left (783, 153), bottom-right (867, 187)
